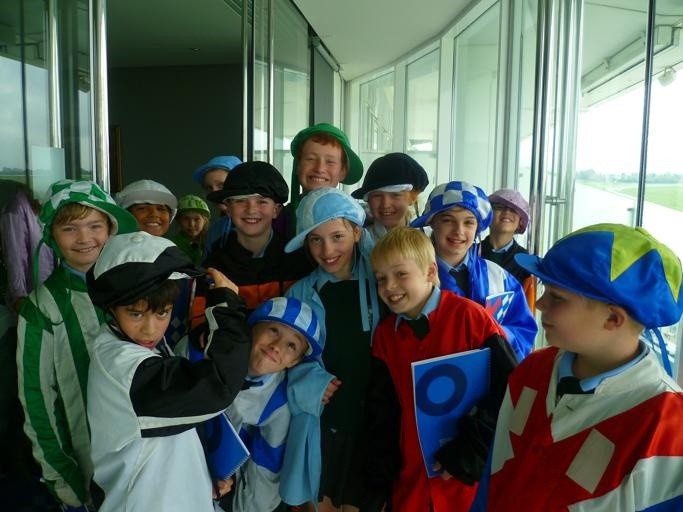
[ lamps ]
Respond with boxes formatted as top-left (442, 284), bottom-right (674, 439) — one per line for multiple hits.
top-left (657, 66), bottom-right (677, 88)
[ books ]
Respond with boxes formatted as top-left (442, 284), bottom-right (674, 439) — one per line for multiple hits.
top-left (410, 347), bottom-right (493, 479)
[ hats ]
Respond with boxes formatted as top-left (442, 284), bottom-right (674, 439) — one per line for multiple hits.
top-left (489, 190), bottom-right (530, 234)
top-left (351, 152), bottom-right (429, 199)
top-left (248, 296), bottom-right (323, 361)
top-left (409, 181), bottom-right (494, 235)
top-left (86, 230), bottom-right (209, 305)
top-left (116, 179), bottom-right (178, 224)
top-left (513, 222), bottom-right (683, 327)
top-left (207, 161), bottom-right (288, 203)
top-left (178, 194), bottom-right (210, 217)
top-left (39, 179), bottom-right (139, 248)
top-left (284, 188), bottom-right (366, 253)
top-left (191, 154), bottom-right (243, 178)
top-left (291, 123), bottom-right (363, 184)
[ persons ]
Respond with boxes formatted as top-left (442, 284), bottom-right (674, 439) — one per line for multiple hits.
top-left (115, 122), bottom-right (538, 363)
top-left (282, 185), bottom-right (398, 511)
top-left (14, 179), bottom-right (115, 511)
top-left (2, 177), bottom-right (60, 332)
top-left (357, 224), bottom-right (518, 512)
top-left (483, 222), bottom-right (683, 511)
top-left (173, 294), bottom-right (326, 512)
top-left (83, 230), bottom-right (253, 512)
